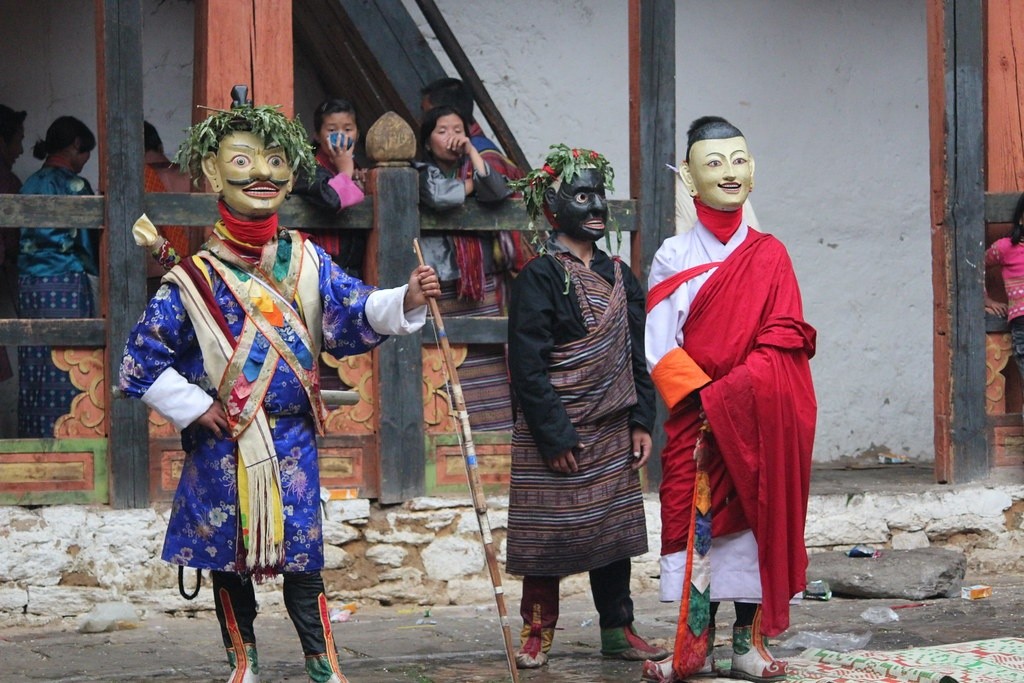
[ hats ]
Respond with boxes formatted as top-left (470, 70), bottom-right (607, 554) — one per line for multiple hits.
top-left (0, 103), bottom-right (27, 135)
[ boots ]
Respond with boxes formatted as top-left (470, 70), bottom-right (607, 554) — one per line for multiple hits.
top-left (729, 602), bottom-right (788, 682)
top-left (589, 558), bottom-right (669, 661)
top-left (514, 575), bottom-right (562, 669)
top-left (210, 571), bottom-right (259, 683)
top-left (641, 602), bottom-right (717, 683)
top-left (283, 571), bottom-right (349, 683)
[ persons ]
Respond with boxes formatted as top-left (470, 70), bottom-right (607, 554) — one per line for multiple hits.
top-left (643, 117), bottom-right (819, 682)
top-left (116, 83), bottom-right (443, 683)
top-left (501, 140), bottom-right (670, 668)
top-left (982, 192), bottom-right (1024, 391)
top-left (0, 77), bottom-right (516, 438)
